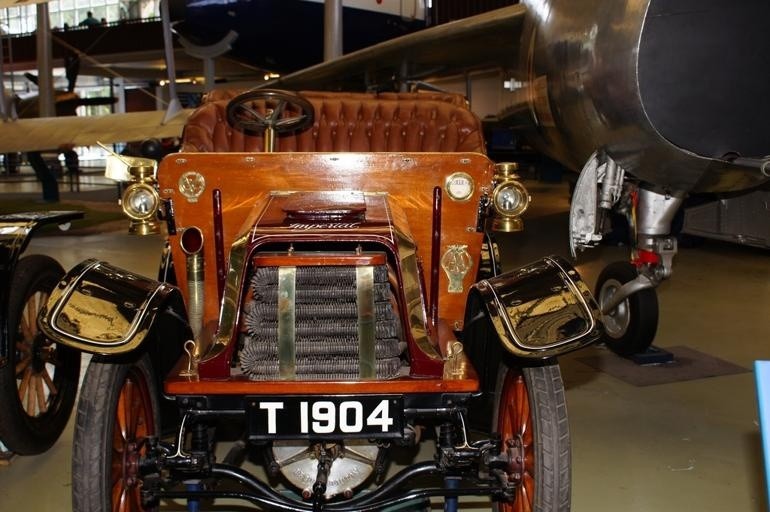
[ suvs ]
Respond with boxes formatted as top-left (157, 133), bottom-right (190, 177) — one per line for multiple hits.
top-left (38, 88), bottom-right (608, 511)
top-left (1, 208), bottom-right (82, 469)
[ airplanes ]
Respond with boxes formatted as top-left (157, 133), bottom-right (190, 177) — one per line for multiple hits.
top-left (0, 0), bottom-right (199, 156)
top-left (251, 1), bottom-right (770, 356)
